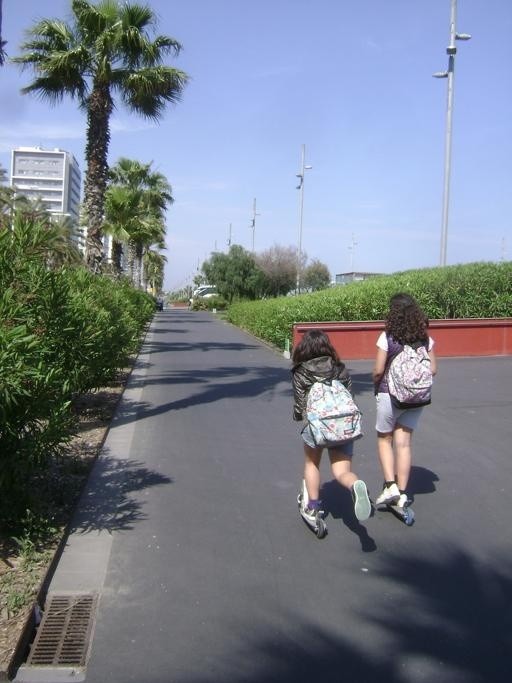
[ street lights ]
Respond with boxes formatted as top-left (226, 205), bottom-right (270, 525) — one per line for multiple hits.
top-left (432, 1), bottom-right (472, 268)
top-left (294, 142), bottom-right (313, 295)
top-left (195, 194), bottom-right (262, 284)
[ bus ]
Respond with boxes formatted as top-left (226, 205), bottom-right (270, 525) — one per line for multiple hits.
top-left (190, 282), bottom-right (221, 307)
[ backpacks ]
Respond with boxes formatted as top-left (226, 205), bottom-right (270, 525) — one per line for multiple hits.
top-left (388, 328), bottom-right (433, 409)
top-left (290, 361), bottom-right (363, 447)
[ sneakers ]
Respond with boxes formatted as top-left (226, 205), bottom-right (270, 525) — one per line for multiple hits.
top-left (396, 491), bottom-right (409, 509)
top-left (376, 484), bottom-right (400, 508)
top-left (352, 479), bottom-right (372, 521)
top-left (303, 504), bottom-right (318, 521)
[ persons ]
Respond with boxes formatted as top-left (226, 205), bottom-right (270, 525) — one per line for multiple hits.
top-left (370, 292), bottom-right (435, 509)
top-left (290, 331), bottom-right (374, 522)
